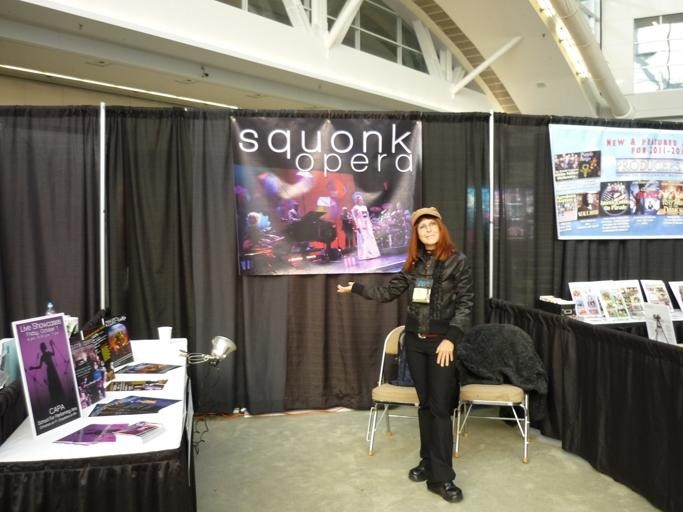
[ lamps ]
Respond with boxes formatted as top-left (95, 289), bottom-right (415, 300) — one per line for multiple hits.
top-left (179, 334), bottom-right (236, 368)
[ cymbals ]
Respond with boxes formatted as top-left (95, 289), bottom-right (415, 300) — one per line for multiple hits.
top-left (369, 207), bottom-right (381, 212)
top-left (382, 203), bottom-right (393, 209)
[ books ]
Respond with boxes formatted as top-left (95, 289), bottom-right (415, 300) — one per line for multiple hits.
top-left (12, 308), bottom-right (185, 446)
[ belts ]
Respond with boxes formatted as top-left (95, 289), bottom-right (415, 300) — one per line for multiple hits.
top-left (416, 332), bottom-right (436, 339)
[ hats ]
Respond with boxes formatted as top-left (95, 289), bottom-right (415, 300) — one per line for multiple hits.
top-left (410, 206), bottom-right (441, 225)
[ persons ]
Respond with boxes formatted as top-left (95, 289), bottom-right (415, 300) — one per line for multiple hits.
top-left (332, 206), bottom-right (475, 502)
top-left (395, 202), bottom-right (402, 213)
top-left (350, 191), bottom-right (380, 259)
top-left (554, 151), bottom-right (599, 180)
top-left (633, 184), bottom-right (647, 215)
top-left (285, 200), bottom-right (309, 261)
top-left (577, 192), bottom-right (598, 219)
top-left (245, 212), bottom-right (277, 247)
top-left (340, 206), bottom-right (354, 249)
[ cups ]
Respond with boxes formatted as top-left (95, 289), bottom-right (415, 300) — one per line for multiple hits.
top-left (158, 326), bottom-right (172, 345)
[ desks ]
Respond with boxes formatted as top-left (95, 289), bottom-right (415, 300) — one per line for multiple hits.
top-left (0, 337), bottom-right (198, 511)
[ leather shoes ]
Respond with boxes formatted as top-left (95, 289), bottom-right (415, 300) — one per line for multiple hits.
top-left (408, 459), bottom-right (429, 482)
top-left (426, 479), bottom-right (463, 504)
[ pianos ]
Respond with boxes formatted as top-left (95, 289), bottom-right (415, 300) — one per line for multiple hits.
top-left (264, 211), bottom-right (337, 266)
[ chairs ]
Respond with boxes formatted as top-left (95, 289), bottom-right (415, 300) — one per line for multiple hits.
top-left (363, 324), bottom-right (531, 465)
